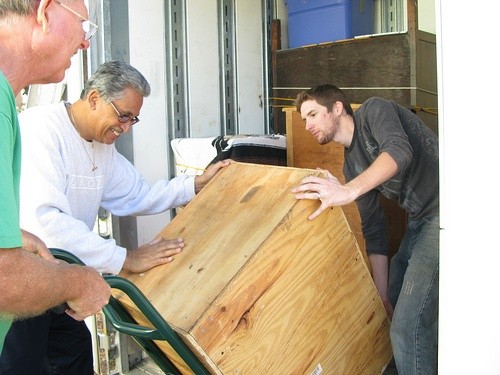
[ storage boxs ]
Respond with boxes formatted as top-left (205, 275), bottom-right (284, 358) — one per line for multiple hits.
top-left (284, 0), bottom-right (374, 48)
top-left (113, 162), bottom-right (393, 375)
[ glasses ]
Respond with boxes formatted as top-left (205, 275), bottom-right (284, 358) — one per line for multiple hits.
top-left (104, 95), bottom-right (139, 125)
top-left (53, 0), bottom-right (98, 41)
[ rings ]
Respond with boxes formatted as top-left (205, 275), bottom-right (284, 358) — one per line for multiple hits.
top-left (317, 193), bottom-right (321, 200)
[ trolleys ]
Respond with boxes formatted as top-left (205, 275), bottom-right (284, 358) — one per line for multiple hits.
top-left (47, 248), bottom-right (212, 375)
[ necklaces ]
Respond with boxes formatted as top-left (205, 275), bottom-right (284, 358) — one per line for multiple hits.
top-left (68, 102), bottom-right (98, 173)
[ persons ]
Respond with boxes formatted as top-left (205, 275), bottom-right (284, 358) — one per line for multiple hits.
top-left (0, 0), bottom-right (113, 358)
top-left (0, 61), bottom-right (229, 375)
top-left (290, 85), bottom-right (439, 375)
